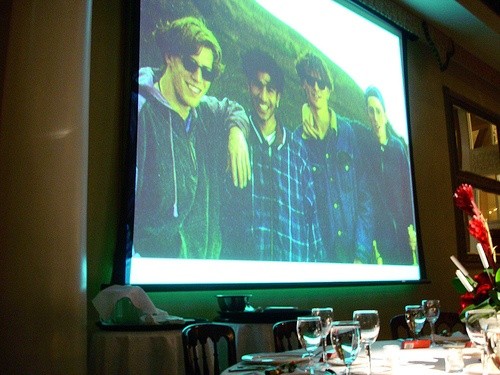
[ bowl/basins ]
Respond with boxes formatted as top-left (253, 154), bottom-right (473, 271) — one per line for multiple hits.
top-left (215, 295), bottom-right (252, 312)
top-left (100, 296), bottom-right (141, 326)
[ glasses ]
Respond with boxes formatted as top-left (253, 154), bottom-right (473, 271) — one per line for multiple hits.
top-left (178, 54), bottom-right (218, 82)
top-left (306, 75), bottom-right (329, 90)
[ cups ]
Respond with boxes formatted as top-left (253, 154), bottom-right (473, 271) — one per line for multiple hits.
top-left (444, 345), bottom-right (464, 373)
top-left (383, 344), bottom-right (400, 366)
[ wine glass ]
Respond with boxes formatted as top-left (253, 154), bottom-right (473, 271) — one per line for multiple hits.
top-left (422, 299), bottom-right (443, 348)
top-left (312, 307), bottom-right (334, 365)
top-left (465, 309), bottom-right (500, 375)
top-left (297, 316), bottom-right (322, 374)
top-left (330, 321), bottom-right (361, 375)
top-left (353, 310), bottom-right (380, 375)
top-left (405, 306), bottom-right (426, 340)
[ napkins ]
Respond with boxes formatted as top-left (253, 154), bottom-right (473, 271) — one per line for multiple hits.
top-left (403, 341), bottom-right (430, 349)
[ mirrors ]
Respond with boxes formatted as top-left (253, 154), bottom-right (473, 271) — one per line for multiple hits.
top-left (443, 85), bottom-right (500, 270)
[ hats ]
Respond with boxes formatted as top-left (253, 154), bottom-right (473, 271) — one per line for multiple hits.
top-left (365, 86), bottom-right (383, 105)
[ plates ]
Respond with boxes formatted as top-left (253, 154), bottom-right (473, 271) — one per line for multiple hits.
top-left (241, 352), bottom-right (313, 363)
top-left (328, 355), bottom-right (369, 365)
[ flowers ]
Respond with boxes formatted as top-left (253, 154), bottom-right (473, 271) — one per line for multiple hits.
top-left (452, 183), bottom-right (500, 322)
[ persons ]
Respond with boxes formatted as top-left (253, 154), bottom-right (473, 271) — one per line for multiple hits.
top-left (131, 15), bottom-right (252, 258)
top-left (214, 47), bottom-right (324, 263)
top-left (291, 51), bottom-right (374, 265)
top-left (301, 87), bottom-right (414, 267)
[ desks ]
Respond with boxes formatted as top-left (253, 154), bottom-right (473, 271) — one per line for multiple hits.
top-left (221, 337), bottom-right (483, 374)
top-left (207, 322), bottom-right (299, 375)
top-left (89, 329), bottom-right (215, 375)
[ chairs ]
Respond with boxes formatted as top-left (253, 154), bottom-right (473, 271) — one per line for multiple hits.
top-left (273, 320), bottom-right (332, 353)
top-left (391, 312), bottom-right (461, 340)
top-left (182, 323), bottom-right (236, 375)
